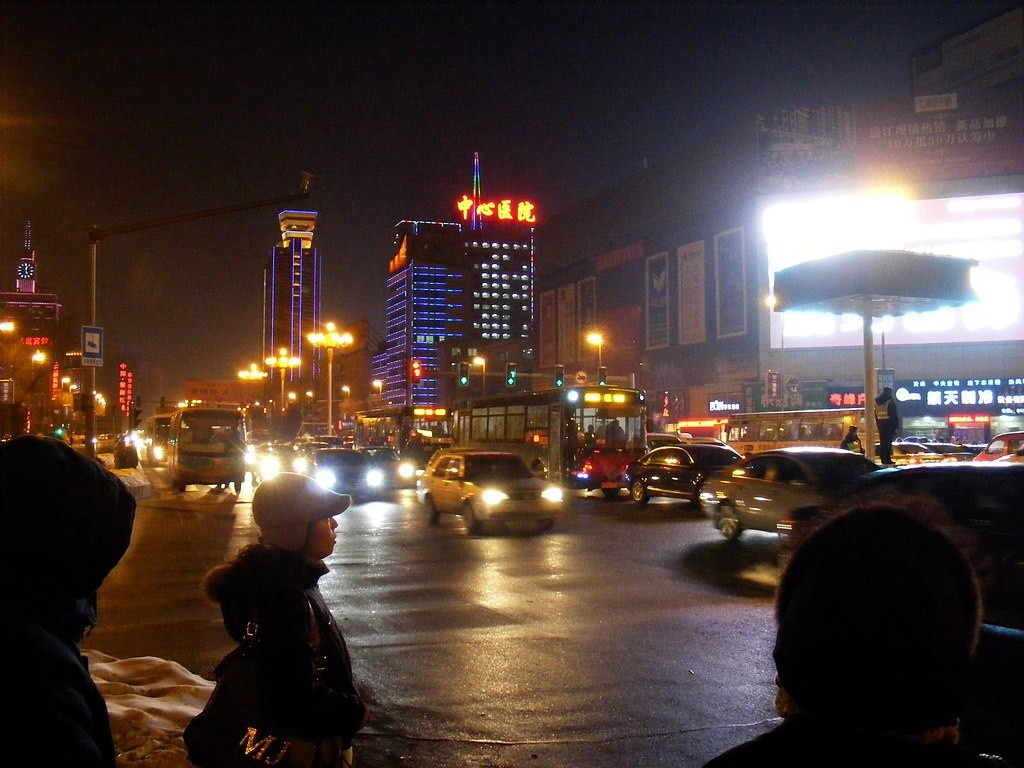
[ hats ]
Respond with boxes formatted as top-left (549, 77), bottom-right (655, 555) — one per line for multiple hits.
top-left (252, 472), bottom-right (351, 550)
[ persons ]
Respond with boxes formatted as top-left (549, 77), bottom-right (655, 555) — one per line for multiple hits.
top-left (604, 419), bottom-right (627, 444)
top-left (433, 422), bottom-right (444, 435)
top-left (584, 424), bottom-right (596, 440)
top-left (894, 436), bottom-right (979, 445)
top-left (183, 471), bottom-right (371, 768)
top-left (840, 425), bottom-right (865, 456)
top-left (0, 428), bottom-right (138, 768)
top-left (781, 415), bottom-right (844, 442)
top-left (698, 492), bottom-right (1024, 768)
top-left (873, 386), bottom-right (900, 464)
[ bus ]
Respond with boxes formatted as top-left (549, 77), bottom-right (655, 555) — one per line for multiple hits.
top-left (470, 385), bottom-right (648, 498)
top-left (136, 415), bottom-right (171, 463)
top-left (169, 408), bottom-right (247, 493)
top-left (724, 408), bottom-right (881, 455)
top-left (352, 405), bottom-right (456, 461)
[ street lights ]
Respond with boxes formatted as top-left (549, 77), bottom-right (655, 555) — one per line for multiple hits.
top-left (308, 322), bottom-right (382, 436)
top-left (264, 347), bottom-right (302, 415)
top-left (237, 364), bottom-right (268, 403)
top-left (587, 333), bottom-right (602, 366)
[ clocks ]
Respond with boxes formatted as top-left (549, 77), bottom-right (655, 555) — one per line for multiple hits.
top-left (17, 263), bottom-right (34, 278)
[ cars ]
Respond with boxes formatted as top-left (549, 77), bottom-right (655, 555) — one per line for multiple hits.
top-left (701, 448), bottom-right (881, 545)
top-left (646, 433), bottom-right (725, 446)
top-left (627, 447), bottom-right (746, 505)
top-left (244, 440), bottom-right (413, 500)
top-left (896, 431), bottom-right (1024, 463)
top-left (414, 447), bottom-right (567, 534)
top-left (775, 446), bottom-right (1024, 631)
top-left (67, 433), bottom-right (117, 454)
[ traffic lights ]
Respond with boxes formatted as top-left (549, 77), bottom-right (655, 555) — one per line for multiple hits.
top-left (411, 361), bottom-right (608, 388)
top-left (58, 428), bottom-right (62, 435)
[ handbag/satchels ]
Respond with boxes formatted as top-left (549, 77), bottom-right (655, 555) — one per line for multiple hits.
top-left (182, 595), bottom-right (342, 768)
